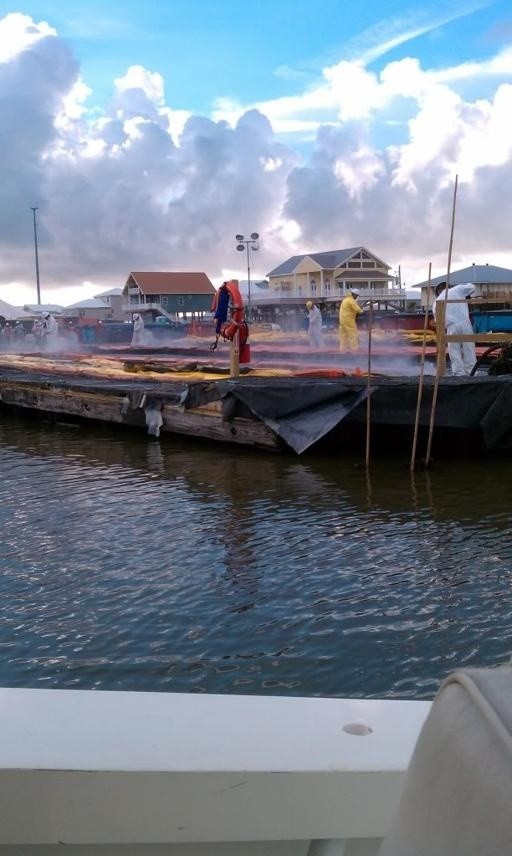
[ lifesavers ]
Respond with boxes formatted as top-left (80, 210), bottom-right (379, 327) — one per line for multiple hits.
top-left (211, 281), bottom-right (243, 339)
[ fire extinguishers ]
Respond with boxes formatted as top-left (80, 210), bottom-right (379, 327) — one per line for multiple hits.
top-left (239, 319), bottom-right (250, 363)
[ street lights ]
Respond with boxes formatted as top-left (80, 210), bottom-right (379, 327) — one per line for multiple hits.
top-left (236, 233), bottom-right (259, 324)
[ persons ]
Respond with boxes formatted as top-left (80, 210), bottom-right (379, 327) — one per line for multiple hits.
top-left (131, 313), bottom-right (144, 347)
top-left (5, 312), bottom-right (58, 344)
top-left (306, 301), bottom-right (325, 348)
top-left (339, 289), bottom-right (364, 351)
top-left (431, 282), bottom-right (478, 376)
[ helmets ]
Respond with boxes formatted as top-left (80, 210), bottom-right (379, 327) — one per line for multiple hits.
top-left (132, 314), bottom-right (139, 320)
top-left (350, 288), bottom-right (361, 296)
top-left (40, 311), bottom-right (50, 319)
top-left (434, 281), bottom-right (450, 297)
top-left (306, 301), bottom-right (313, 310)
top-left (4, 319), bottom-right (39, 326)
